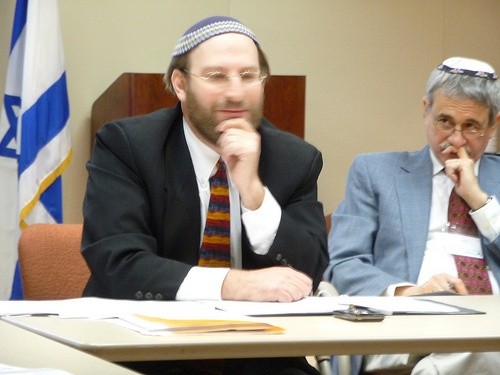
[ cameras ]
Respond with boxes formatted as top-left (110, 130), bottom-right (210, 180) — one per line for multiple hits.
top-left (333, 305), bottom-right (385, 322)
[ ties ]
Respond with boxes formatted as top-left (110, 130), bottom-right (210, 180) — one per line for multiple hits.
top-left (446, 184), bottom-right (493, 296)
top-left (199, 157), bottom-right (231, 268)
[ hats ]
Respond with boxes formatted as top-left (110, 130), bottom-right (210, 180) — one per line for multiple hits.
top-left (172, 16), bottom-right (261, 57)
top-left (438, 57), bottom-right (500, 81)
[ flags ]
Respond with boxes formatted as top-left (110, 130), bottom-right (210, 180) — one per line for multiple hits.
top-left (0, 0), bottom-right (74, 301)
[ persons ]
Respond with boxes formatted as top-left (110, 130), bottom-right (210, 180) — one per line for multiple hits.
top-left (324, 57), bottom-right (500, 375)
top-left (80, 16), bottom-right (330, 375)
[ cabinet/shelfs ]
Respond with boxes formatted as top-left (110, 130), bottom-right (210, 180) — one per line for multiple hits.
top-left (89, 74), bottom-right (303, 169)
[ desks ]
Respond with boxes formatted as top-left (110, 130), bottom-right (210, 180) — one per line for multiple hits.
top-left (0, 319), bottom-right (143, 375)
top-left (0, 294), bottom-right (500, 359)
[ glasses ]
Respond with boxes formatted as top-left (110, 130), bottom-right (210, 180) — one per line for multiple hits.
top-left (182, 68), bottom-right (269, 91)
top-left (428, 105), bottom-right (491, 141)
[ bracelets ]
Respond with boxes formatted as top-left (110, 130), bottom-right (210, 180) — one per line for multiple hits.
top-left (472, 196), bottom-right (492, 212)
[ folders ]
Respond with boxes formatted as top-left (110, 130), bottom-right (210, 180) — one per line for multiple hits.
top-left (196, 296), bottom-right (486, 316)
top-left (133, 312), bottom-right (285, 333)
